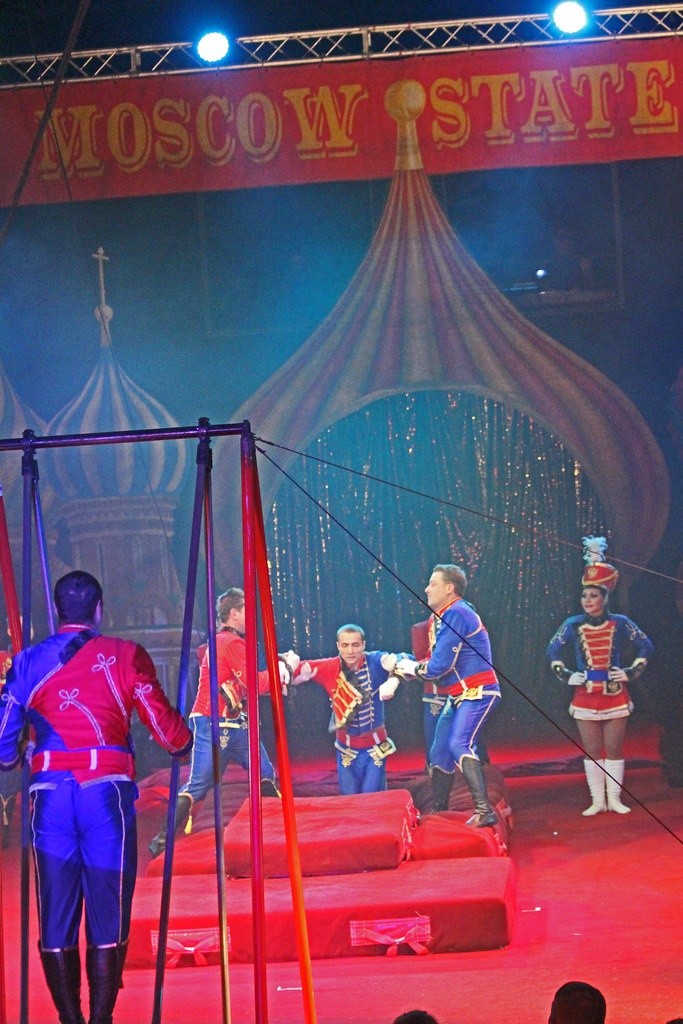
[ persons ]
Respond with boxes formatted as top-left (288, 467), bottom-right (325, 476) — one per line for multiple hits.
top-left (148, 590), bottom-right (318, 857)
top-left (546, 537), bottom-right (653, 814)
top-left (381, 564), bottom-right (501, 827)
top-left (0, 570), bottom-right (194, 1024)
top-left (394, 1009), bottom-right (437, 1024)
top-left (279, 625), bottom-right (417, 794)
top-left (548, 981), bottom-right (606, 1024)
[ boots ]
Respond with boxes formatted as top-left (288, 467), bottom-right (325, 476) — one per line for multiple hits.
top-left (454, 753), bottom-right (497, 828)
top-left (38, 939), bottom-right (87, 1024)
top-left (425, 761), bottom-right (457, 812)
top-left (83, 938), bottom-right (130, 1024)
top-left (604, 759), bottom-right (631, 814)
top-left (261, 770), bottom-right (285, 798)
top-left (583, 758), bottom-right (608, 816)
top-left (149, 793), bottom-right (194, 857)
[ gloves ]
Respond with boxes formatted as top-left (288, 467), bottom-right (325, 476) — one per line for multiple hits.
top-left (567, 672), bottom-right (586, 685)
top-left (282, 651), bottom-right (300, 673)
top-left (279, 661), bottom-right (290, 685)
top-left (397, 659), bottom-right (419, 676)
top-left (380, 653), bottom-right (397, 672)
top-left (379, 677), bottom-right (400, 701)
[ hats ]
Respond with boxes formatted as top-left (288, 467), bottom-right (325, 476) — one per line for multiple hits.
top-left (582, 535), bottom-right (619, 593)
